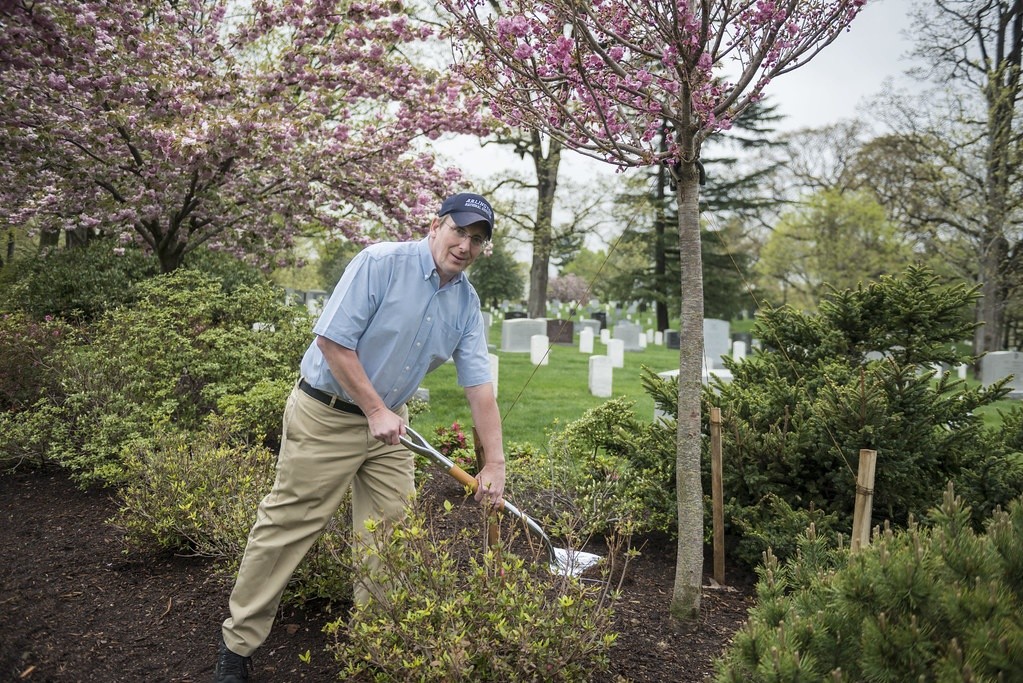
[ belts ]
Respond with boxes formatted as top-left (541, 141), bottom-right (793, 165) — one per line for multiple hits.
top-left (297, 373), bottom-right (365, 417)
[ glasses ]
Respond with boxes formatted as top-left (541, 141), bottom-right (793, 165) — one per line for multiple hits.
top-left (443, 221), bottom-right (485, 246)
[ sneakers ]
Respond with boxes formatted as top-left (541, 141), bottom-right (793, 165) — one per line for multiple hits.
top-left (216, 639), bottom-right (253, 683)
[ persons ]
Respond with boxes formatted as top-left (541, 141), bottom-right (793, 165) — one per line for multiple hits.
top-left (215, 193), bottom-right (505, 683)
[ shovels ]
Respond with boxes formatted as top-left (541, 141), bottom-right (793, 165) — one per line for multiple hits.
top-left (399, 425), bottom-right (604, 577)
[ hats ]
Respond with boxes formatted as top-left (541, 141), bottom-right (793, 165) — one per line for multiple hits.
top-left (439, 193), bottom-right (493, 240)
